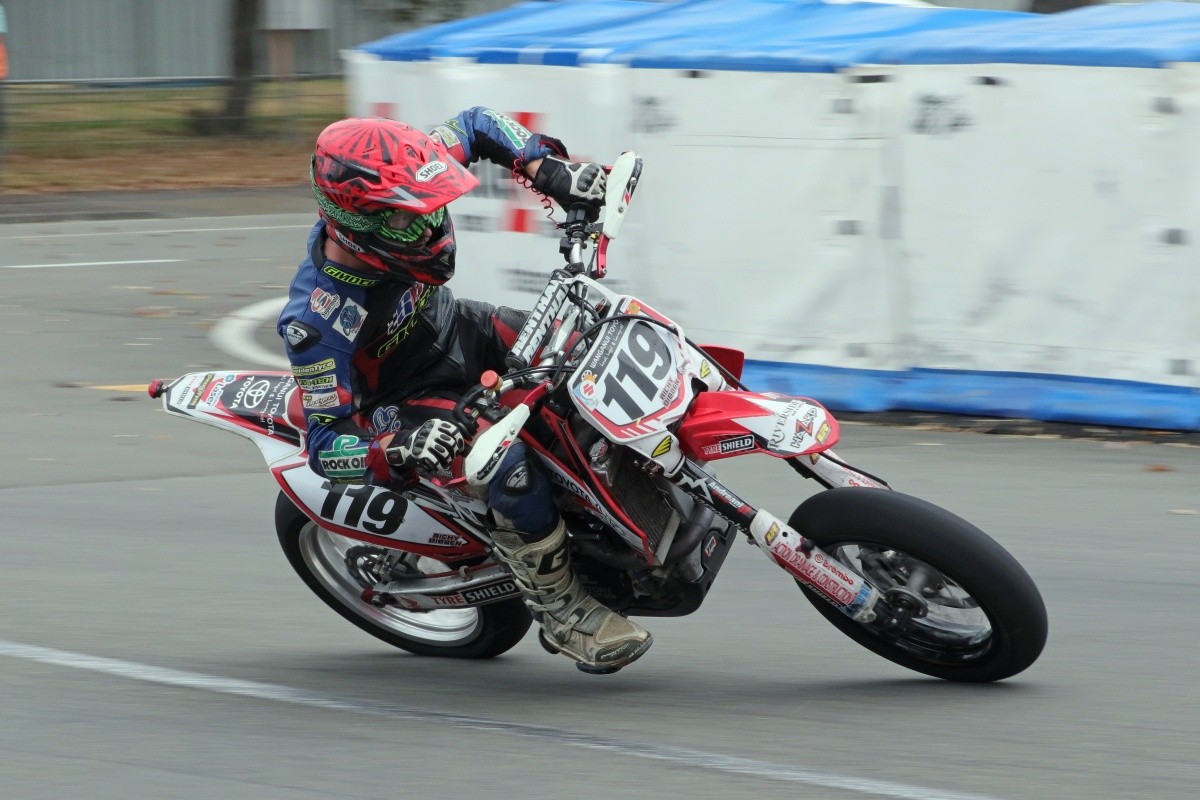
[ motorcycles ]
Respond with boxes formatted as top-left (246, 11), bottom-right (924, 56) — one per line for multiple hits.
top-left (144, 149), bottom-right (1049, 686)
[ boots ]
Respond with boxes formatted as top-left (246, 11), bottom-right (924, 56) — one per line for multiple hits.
top-left (493, 515), bottom-right (654, 667)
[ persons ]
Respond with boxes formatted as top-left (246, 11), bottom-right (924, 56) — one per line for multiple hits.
top-left (274, 105), bottom-right (654, 669)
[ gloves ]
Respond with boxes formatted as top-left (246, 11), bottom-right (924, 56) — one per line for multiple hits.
top-left (532, 154), bottom-right (608, 224)
top-left (385, 418), bottom-right (465, 480)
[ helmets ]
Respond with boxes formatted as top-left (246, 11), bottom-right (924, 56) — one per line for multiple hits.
top-left (308, 117), bottom-right (479, 287)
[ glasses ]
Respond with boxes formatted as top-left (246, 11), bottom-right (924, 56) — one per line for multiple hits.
top-left (379, 207), bottom-right (444, 242)
